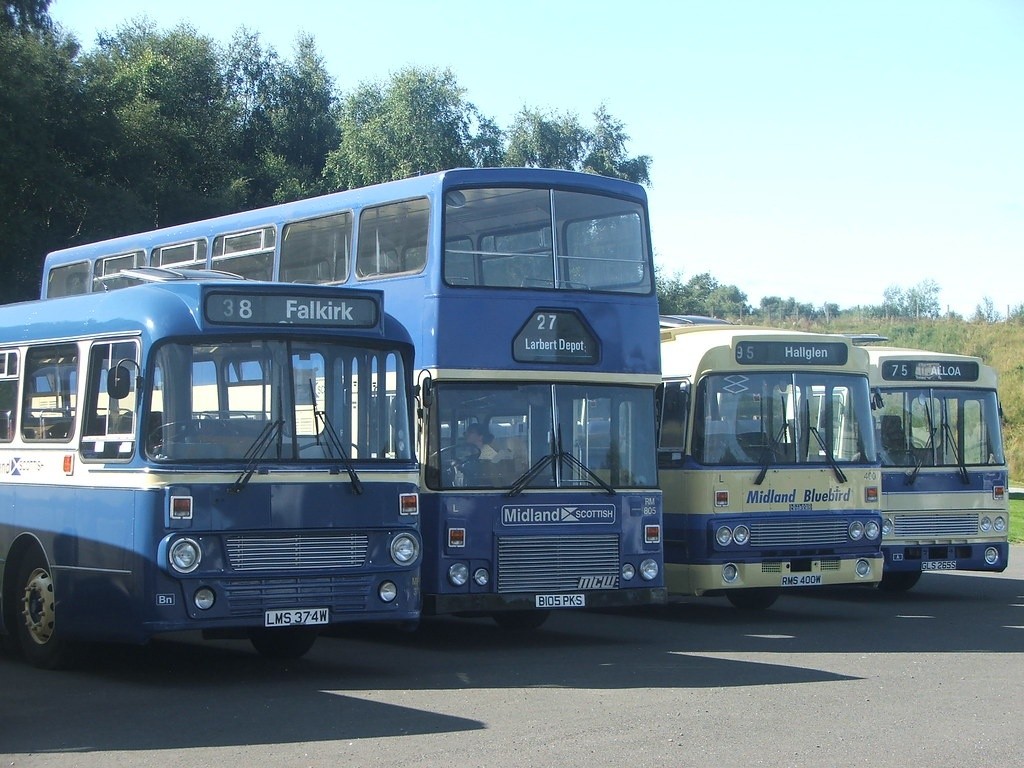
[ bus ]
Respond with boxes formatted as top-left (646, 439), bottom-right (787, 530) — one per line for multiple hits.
top-left (657, 315), bottom-right (883, 611)
top-left (0, 267), bottom-right (432, 672)
top-left (774, 334), bottom-right (1009, 593)
top-left (31, 167), bottom-right (666, 640)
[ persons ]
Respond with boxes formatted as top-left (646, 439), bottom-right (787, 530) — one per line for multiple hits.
top-left (463, 423), bottom-right (498, 459)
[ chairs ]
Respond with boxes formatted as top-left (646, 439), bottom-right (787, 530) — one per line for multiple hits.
top-left (492, 436), bottom-right (530, 472)
top-left (879, 415), bottom-right (913, 450)
top-left (725, 431), bottom-right (773, 462)
top-left (23, 407), bottom-right (246, 438)
